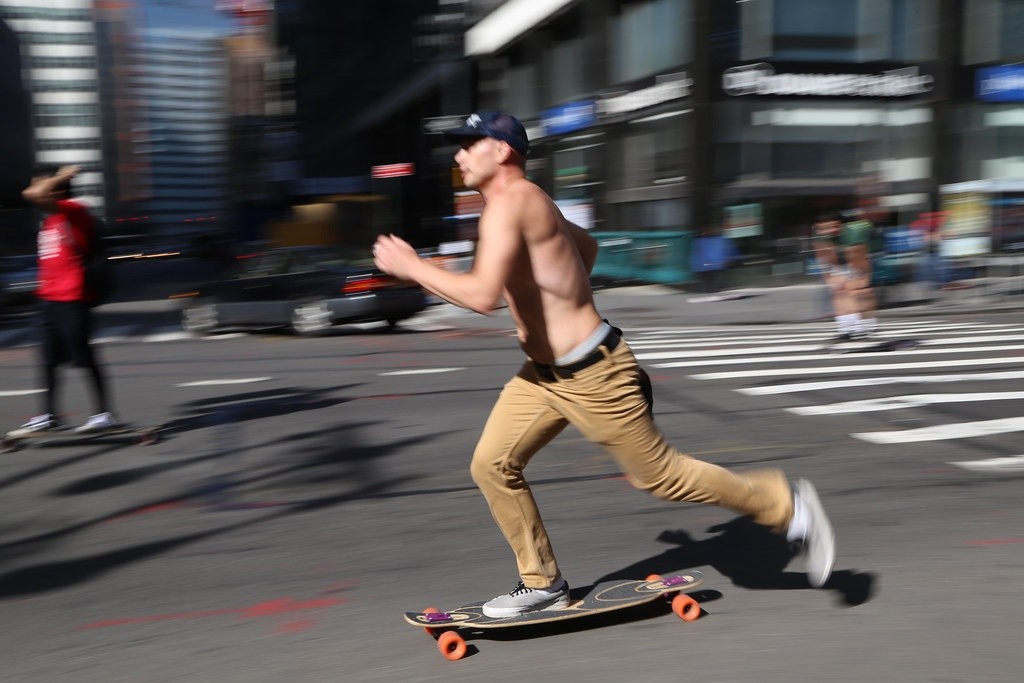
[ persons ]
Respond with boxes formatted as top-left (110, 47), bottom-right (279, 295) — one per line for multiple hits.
top-left (6, 160), bottom-right (118, 439)
top-left (371, 111), bottom-right (838, 619)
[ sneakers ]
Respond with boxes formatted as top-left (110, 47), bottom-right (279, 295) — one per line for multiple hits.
top-left (482, 582), bottom-right (570, 618)
top-left (792, 478), bottom-right (834, 589)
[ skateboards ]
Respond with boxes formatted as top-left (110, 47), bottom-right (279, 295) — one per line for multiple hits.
top-left (401, 568), bottom-right (702, 660)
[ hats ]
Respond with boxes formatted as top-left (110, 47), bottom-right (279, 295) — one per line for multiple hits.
top-left (441, 108), bottom-right (529, 157)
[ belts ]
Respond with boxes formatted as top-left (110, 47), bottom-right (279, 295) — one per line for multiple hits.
top-left (532, 327), bottom-right (621, 379)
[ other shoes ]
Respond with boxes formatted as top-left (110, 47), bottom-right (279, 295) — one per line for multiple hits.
top-left (74, 411), bottom-right (117, 433)
top-left (6, 414), bottom-right (56, 436)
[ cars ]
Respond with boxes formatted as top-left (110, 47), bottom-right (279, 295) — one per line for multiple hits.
top-left (174, 242), bottom-right (427, 334)
